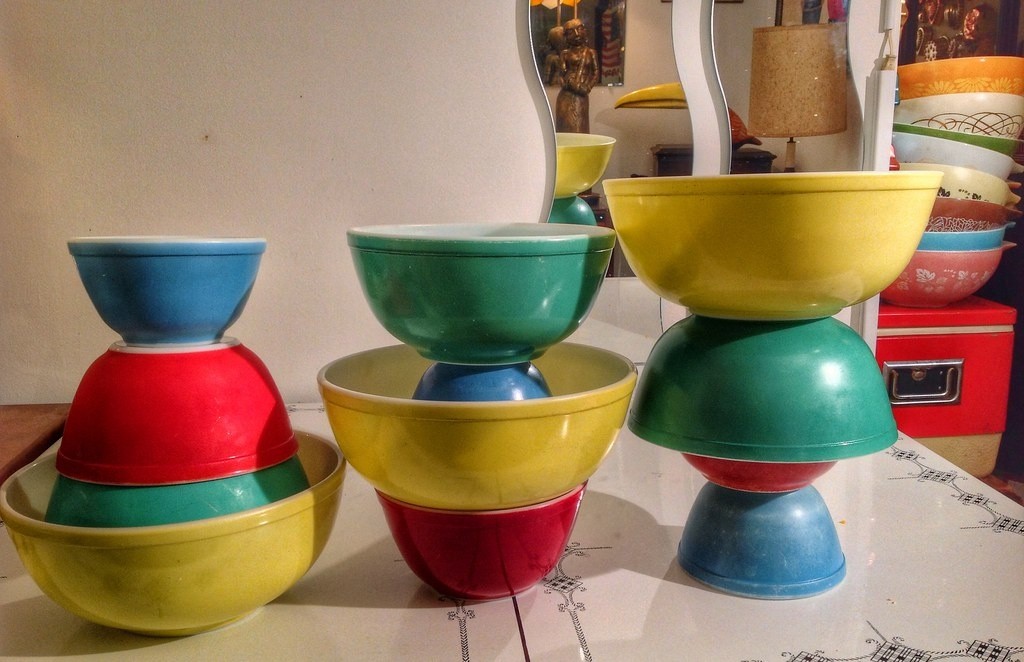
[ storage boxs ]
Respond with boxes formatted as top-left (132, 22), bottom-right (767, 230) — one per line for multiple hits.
top-left (873, 297), bottom-right (1018, 439)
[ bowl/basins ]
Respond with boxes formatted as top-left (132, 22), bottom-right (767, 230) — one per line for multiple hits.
top-left (412, 361), bottom-right (554, 403)
top-left (554, 131), bottom-right (617, 199)
top-left (51, 338), bottom-right (299, 488)
top-left (340, 224), bottom-right (619, 370)
top-left (45, 455), bottom-right (311, 529)
top-left (881, 56), bottom-right (1024, 309)
top-left (64, 237), bottom-right (268, 344)
top-left (677, 483), bottom-right (847, 602)
top-left (373, 480), bottom-right (591, 601)
top-left (545, 195), bottom-right (601, 225)
top-left (316, 343), bottom-right (639, 509)
top-left (602, 168), bottom-right (943, 320)
top-left (677, 448), bottom-right (840, 494)
top-left (626, 310), bottom-right (900, 462)
top-left (0, 427), bottom-right (346, 639)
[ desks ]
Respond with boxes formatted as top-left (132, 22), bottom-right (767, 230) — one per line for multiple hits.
top-left (0, 404), bottom-right (1023, 662)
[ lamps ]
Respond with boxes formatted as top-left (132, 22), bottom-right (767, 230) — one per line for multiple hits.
top-left (745, 20), bottom-right (848, 174)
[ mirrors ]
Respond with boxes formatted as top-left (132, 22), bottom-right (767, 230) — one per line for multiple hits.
top-left (536, 0), bottom-right (625, 87)
top-left (514, 0), bottom-right (889, 280)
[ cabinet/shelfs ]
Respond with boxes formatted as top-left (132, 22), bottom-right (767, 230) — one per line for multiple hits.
top-left (650, 144), bottom-right (775, 176)
